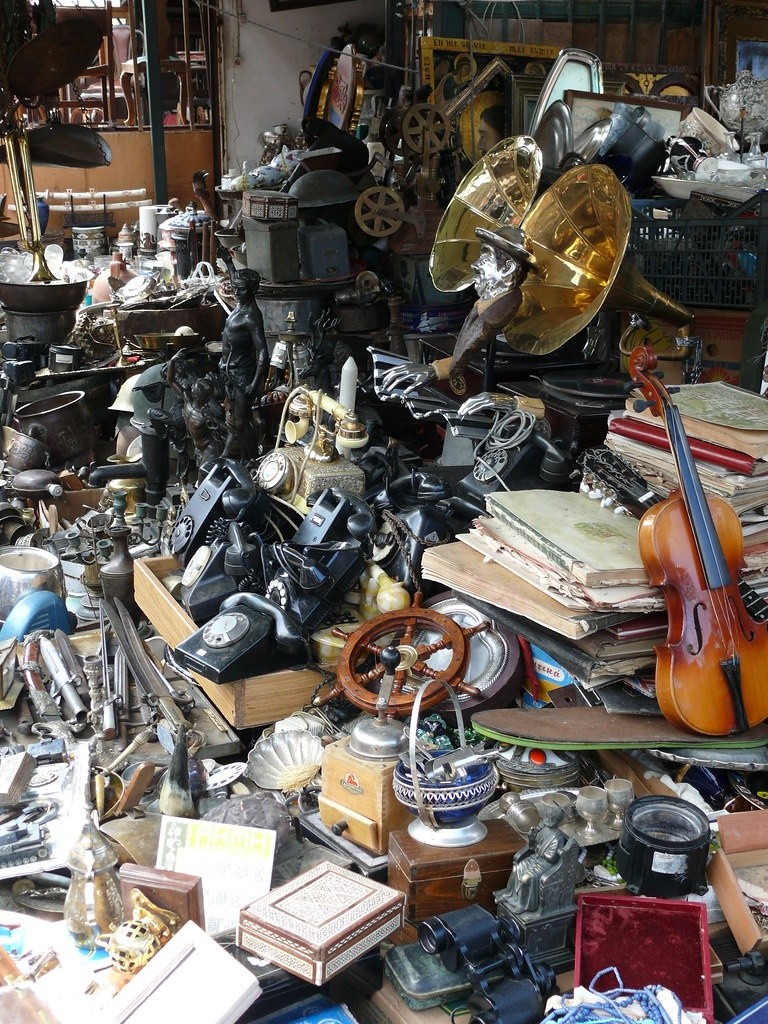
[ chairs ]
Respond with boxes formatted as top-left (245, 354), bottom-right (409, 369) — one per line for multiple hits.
top-left (59, 0), bottom-right (189, 124)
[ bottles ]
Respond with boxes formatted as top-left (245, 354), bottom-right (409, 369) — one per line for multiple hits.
top-left (677, 169), bottom-right (768, 189)
top-left (742, 132), bottom-right (768, 167)
top-left (100, 526), bottom-right (141, 630)
top-left (666, 135), bottom-right (717, 172)
top-left (716, 132), bottom-right (741, 169)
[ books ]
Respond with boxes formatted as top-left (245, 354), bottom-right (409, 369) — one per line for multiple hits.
top-left (451, 490), bottom-right (665, 615)
top-left (607, 380), bottom-right (768, 601)
top-left (420, 542), bottom-right (599, 642)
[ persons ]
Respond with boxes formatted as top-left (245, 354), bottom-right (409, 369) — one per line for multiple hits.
top-left (380, 226), bottom-right (537, 396)
top-left (132, 268), bottom-right (270, 485)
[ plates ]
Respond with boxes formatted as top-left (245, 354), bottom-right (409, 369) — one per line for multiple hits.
top-left (651, 174), bottom-right (768, 202)
top-left (476, 787), bottom-right (621, 848)
top-left (574, 118), bottom-right (613, 164)
top-left (535, 100), bottom-right (574, 169)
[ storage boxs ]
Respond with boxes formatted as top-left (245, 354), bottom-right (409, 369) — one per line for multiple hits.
top-left (133, 557), bottom-right (338, 730)
top-left (236, 860), bottom-right (405, 987)
top-left (388, 819), bottom-right (525, 922)
top-left (215, 931), bottom-right (384, 1024)
top-left (706, 810), bottom-right (768, 956)
top-left (620, 198), bottom-right (768, 385)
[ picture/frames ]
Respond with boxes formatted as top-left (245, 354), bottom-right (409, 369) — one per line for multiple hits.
top-left (420, 38), bottom-right (559, 169)
top-left (511, 74), bottom-right (625, 134)
top-left (563, 89), bottom-right (689, 139)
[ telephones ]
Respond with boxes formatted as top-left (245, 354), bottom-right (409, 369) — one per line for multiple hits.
top-left (255, 381), bottom-right (370, 514)
top-left (170, 457), bottom-right (264, 564)
top-left (376, 469), bottom-right (456, 601)
top-left (174, 520), bottom-right (264, 621)
top-left (266, 486), bottom-right (376, 625)
top-left (459, 426), bottom-right (575, 510)
top-left (173, 590), bottom-right (300, 684)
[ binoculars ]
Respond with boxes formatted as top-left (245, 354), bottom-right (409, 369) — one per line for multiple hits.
top-left (416, 905), bottom-right (555, 1024)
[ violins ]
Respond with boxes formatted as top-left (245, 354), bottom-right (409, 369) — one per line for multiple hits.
top-left (626, 346), bottom-right (768, 737)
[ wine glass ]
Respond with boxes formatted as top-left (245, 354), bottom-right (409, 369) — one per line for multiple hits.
top-left (576, 786), bottom-right (608, 837)
top-left (499, 791), bottom-right (540, 835)
top-left (604, 778), bottom-right (635, 832)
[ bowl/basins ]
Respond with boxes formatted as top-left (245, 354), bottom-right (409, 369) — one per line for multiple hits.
top-left (14, 391), bottom-right (98, 472)
top-left (679, 107), bottom-right (740, 157)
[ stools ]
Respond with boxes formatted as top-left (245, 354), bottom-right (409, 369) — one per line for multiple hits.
top-left (120, 56), bottom-right (188, 125)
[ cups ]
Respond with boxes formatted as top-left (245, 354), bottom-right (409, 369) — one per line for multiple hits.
top-left (540, 793), bottom-right (570, 826)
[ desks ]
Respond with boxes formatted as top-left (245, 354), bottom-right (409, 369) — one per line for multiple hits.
top-left (176, 51), bottom-right (207, 123)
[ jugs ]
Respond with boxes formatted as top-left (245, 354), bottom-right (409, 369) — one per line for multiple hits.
top-left (64, 817), bottom-right (123, 951)
